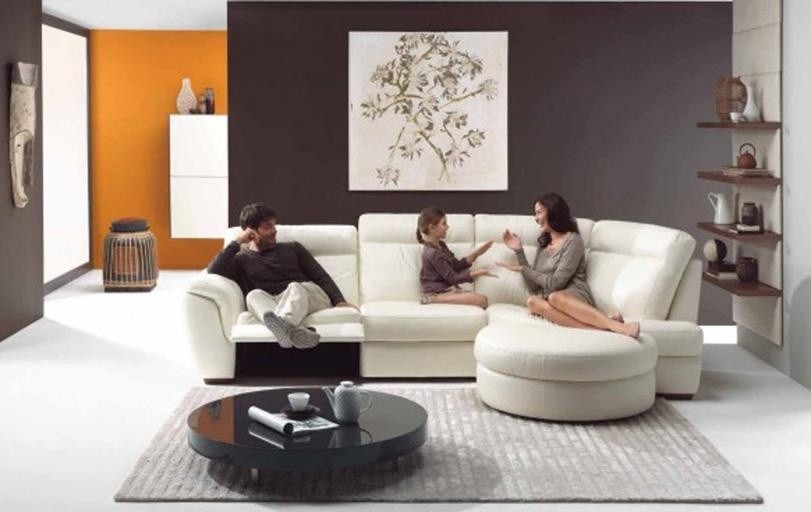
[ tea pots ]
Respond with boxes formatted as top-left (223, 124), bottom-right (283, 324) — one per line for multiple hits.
top-left (736, 143), bottom-right (757, 169)
top-left (707, 191), bottom-right (737, 224)
top-left (322, 380), bottom-right (372, 423)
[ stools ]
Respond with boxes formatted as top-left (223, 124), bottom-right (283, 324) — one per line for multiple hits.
top-left (471, 314), bottom-right (659, 422)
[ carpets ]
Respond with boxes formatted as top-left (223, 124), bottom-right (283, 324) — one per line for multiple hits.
top-left (113, 385), bottom-right (766, 507)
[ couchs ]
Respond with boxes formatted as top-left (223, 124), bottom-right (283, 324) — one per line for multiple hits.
top-left (183, 210), bottom-right (706, 400)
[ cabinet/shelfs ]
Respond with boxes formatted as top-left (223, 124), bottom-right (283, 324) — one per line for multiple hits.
top-left (694, 117), bottom-right (782, 299)
top-left (169, 113), bottom-right (230, 240)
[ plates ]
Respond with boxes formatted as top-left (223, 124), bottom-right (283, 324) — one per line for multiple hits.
top-left (282, 405), bottom-right (318, 420)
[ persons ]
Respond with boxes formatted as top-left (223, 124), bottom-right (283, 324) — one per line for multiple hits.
top-left (496, 192), bottom-right (640, 338)
top-left (416, 206), bottom-right (499, 309)
top-left (208, 203), bottom-right (361, 349)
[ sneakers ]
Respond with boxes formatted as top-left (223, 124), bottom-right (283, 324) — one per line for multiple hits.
top-left (289, 327), bottom-right (321, 352)
top-left (264, 311), bottom-right (293, 353)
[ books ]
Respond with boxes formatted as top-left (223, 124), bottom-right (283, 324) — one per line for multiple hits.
top-left (250, 406), bottom-right (339, 437)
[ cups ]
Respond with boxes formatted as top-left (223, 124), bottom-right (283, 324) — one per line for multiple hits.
top-left (287, 392), bottom-right (310, 411)
top-left (730, 112), bottom-right (743, 123)
